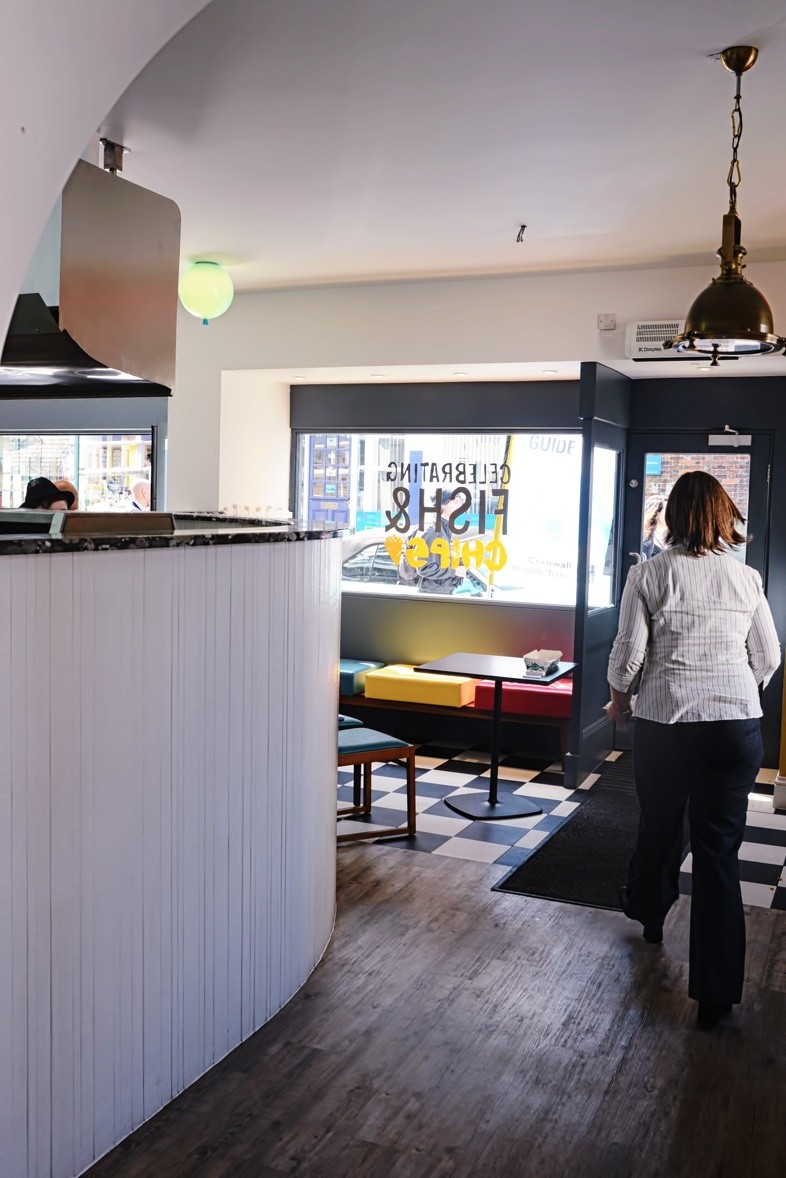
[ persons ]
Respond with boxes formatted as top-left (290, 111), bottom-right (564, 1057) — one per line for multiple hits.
top-left (607, 471), bottom-right (781, 1033)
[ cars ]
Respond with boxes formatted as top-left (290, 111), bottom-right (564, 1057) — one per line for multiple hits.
top-left (341, 525), bottom-right (543, 603)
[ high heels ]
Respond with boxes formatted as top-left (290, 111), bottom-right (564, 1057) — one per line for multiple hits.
top-left (622, 890), bottom-right (665, 942)
top-left (696, 1000), bottom-right (733, 1031)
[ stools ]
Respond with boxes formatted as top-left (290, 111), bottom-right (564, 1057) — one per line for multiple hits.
top-left (337, 714), bottom-right (416, 842)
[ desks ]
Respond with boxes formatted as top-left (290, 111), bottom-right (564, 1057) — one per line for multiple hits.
top-left (414, 653), bottom-right (577, 819)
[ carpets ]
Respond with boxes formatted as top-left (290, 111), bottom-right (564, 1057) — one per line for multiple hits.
top-left (491, 752), bottom-right (690, 911)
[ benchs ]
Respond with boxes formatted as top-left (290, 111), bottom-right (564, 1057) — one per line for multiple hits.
top-left (340, 591), bottom-right (575, 727)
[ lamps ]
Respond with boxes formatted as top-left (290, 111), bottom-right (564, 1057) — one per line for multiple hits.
top-left (178, 261), bottom-right (233, 325)
top-left (664, 46), bottom-right (785, 366)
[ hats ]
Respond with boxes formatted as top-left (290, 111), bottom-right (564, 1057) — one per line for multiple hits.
top-left (20, 477), bottom-right (75, 510)
